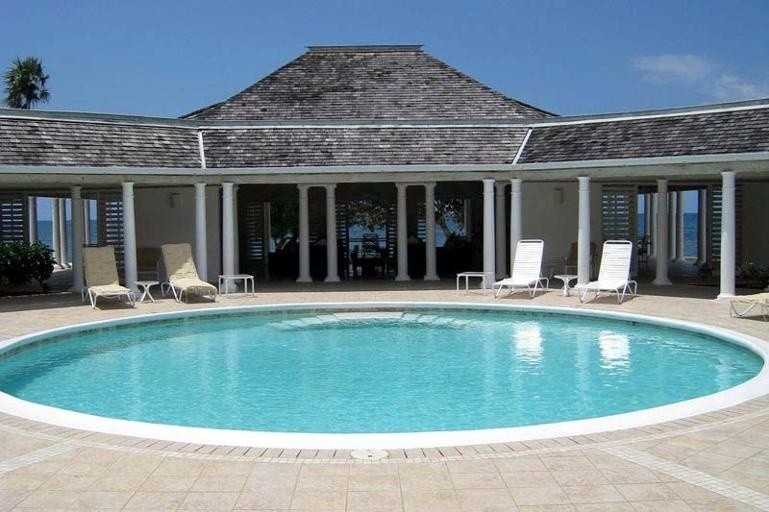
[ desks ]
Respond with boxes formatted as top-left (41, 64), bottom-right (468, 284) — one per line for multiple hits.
top-left (456, 271), bottom-right (494, 292)
top-left (554, 274), bottom-right (578, 297)
top-left (135, 280), bottom-right (160, 304)
top-left (217, 273), bottom-right (255, 298)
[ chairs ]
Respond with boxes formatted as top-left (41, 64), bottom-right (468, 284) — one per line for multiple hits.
top-left (160, 242), bottom-right (217, 303)
top-left (492, 238), bottom-right (549, 298)
top-left (80, 246), bottom-right (137, 310)
top-left (574, 240), bottom-right (637, 305)
